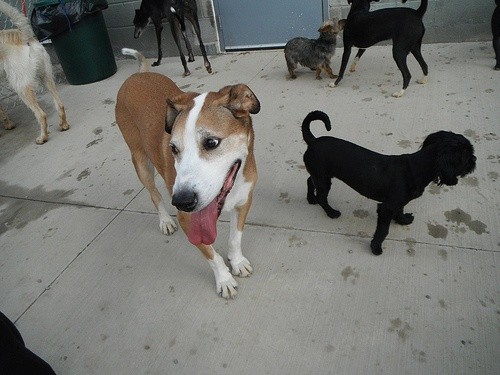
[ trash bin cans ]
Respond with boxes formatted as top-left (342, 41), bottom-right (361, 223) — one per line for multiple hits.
top-left (29, 1), bottom-right (119, 87)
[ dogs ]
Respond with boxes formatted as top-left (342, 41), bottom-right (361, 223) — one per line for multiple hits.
top-left (132, 0), bottom-right (214, 77)
top-left (301, 110), bottom-right (477, 256)
top-left (284, 16), bottom-right (347, 80)
top-left (115, 47), bottom-right (261, 301)
top-left (0, 0), bottom-right (71, 145)
top-left (491, 0), bottom-right (500, 70)
top-left (328, 0), bottom-right (429, 97)
